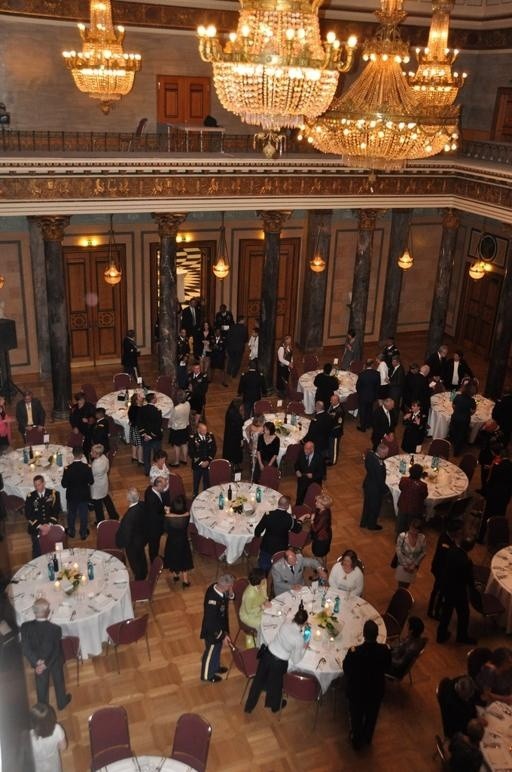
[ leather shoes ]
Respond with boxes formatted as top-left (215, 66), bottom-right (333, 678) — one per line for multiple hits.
top-left (64, 529), bottom-right (74, 535)
top-left (368, 525), bottom-right (382, 530)
top-left (59, 694), bottom-right (70, 709)
top-left (272, 700), bottom-right (286, 712)
top-left (219, 667), bottom-right (228, 673)
top-left (210, 676), bottom-right (222, 682)
top-left (81, 529), bottom-right (89, 540)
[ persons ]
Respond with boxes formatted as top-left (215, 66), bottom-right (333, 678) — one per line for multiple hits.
top-left (0, 298), bottom-right (511, 772)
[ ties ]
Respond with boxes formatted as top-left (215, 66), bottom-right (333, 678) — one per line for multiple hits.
top-left (290, 566), bottom-right (294, 573)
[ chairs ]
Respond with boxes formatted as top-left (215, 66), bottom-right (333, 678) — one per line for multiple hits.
top-left (168, 458), bottom-right (324, 580)
top-left (0, 425), bottom-right (118, 523)
top-left (0, 520), bottom-right (164, 689)
top-left (87, 706), bottom-right (213, 772)
top-left (68, 372), bottom-right (174, 441)
top-left (226, 550), bottom-right (428, 732)
top-left (435, 647), bottom-right (512, 772)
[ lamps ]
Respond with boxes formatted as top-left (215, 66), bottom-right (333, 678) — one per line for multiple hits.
top-left (468, 217), bottom-right (486, 282)
top-left (310, 214), bottom-right (327, 272)
top-left (397, 222), bottom-right (414, 272)
top-left (61, 0), bottom-right (141, 115)
top-left (212, 211), bottom-right (230, 280)
top-left (104, 214), bottom-right (123, 286)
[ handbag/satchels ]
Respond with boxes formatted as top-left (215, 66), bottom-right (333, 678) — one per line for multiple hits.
top-left (391, 552), bottom-right (405, 568)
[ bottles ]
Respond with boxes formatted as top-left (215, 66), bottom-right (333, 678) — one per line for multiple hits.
top-left (57, 448), bottom-right (62, 466)
top-left (299, 595), bottom-right (340, 641)
top-left (23, 444), bottom-right (33, 463)
top-left (219, 484), bottom-right (261, 510)
top-left (451, 388), bottom-right (456, 401)
top-left (398, 453), bottom-right (438, 473)
top-left (125, 387), bottom-right (129, 401)
top-left (48, 553), bottom-right (94, 582)
top-left (283, 412), bottom-right (296, 425)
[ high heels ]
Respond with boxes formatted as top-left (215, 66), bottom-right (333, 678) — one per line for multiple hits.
top-left (174, 576), bottom-right (179, 583)
top-left (182, 582), bottom-right (190, 588)
